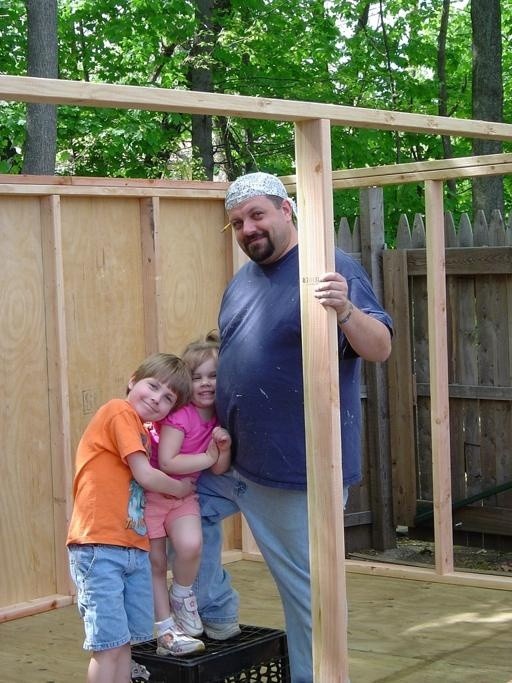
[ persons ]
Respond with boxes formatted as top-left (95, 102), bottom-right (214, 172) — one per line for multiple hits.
top-left (189, 172), bottom-right (395, 683)
top-left (64, 352), bottom-right (193, 682)
top-left (142, 329), bottom-right (232, 659)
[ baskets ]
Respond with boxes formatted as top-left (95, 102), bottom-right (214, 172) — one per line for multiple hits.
top-left (131, 623), bottom-right (291, 683)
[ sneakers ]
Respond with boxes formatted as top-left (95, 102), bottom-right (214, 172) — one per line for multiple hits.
top-left (156, 585), bottom-right (242, 657)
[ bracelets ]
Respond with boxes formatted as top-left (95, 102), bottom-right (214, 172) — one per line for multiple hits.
top-left (337, 302), bottom-right (353, 325)
